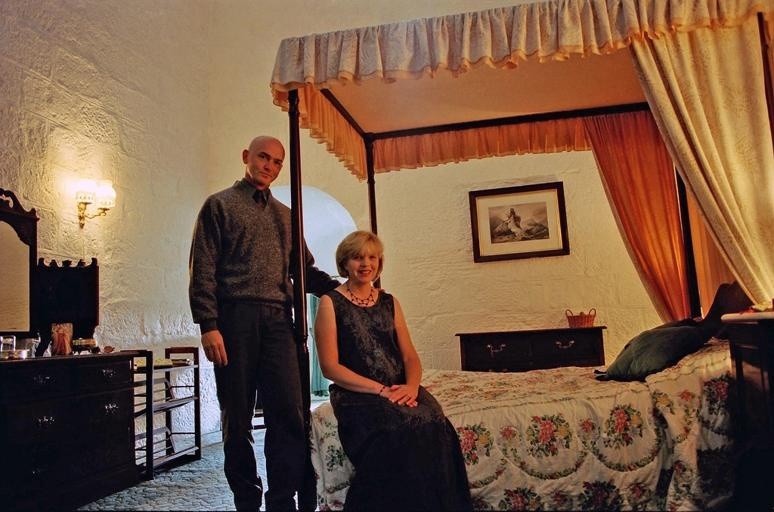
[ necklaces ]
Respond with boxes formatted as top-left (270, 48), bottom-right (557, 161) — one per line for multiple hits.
top-left (345, 281), bottom-right (376, 307)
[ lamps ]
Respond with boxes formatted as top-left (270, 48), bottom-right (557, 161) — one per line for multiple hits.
top-left (75, 179), bottom-right (116, 229)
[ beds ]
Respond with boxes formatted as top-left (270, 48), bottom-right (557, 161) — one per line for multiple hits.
top-left (287, 0), bottom-right (769, 511)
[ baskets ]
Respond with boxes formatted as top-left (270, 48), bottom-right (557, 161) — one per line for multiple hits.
top-left (565, 308), bottom-right (596, 328)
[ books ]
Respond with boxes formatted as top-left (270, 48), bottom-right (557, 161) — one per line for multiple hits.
top-left (154, 396), bottom-right (193, 411)
top-left (172, 359), bottom-right (192, 365)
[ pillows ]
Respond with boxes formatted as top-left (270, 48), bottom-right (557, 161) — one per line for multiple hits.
top-left (594, 318), bottom-right (718, 383)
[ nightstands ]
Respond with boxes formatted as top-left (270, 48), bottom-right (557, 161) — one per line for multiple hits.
top-left (720, 311), bottom-right (774, 447)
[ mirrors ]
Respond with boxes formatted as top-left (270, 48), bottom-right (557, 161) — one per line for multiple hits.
top-left (0, 221), bottom-right (30, 332)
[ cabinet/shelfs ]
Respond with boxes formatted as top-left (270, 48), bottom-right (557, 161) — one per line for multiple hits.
top-left (120, 347), bottom-right (200, 479)
top-left (455, 326), bottom-right (607, 372)
top-left (0, 189), bottom-right (140, 512)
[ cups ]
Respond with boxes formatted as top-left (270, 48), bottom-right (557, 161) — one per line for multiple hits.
top-left (0, 336), bottom-right (15, 350)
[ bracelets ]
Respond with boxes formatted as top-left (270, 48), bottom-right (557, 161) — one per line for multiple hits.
top-left (377, 385), bottom-right (386, 397)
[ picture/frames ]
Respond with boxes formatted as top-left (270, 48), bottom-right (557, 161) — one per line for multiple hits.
top-left (469, 182), bottom-right (570, 262)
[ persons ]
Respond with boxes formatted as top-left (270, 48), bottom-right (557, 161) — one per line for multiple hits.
top-left (190, 134), bottom-right (341, 510)
top-left (314, 231), bottom-right (474, 510)
